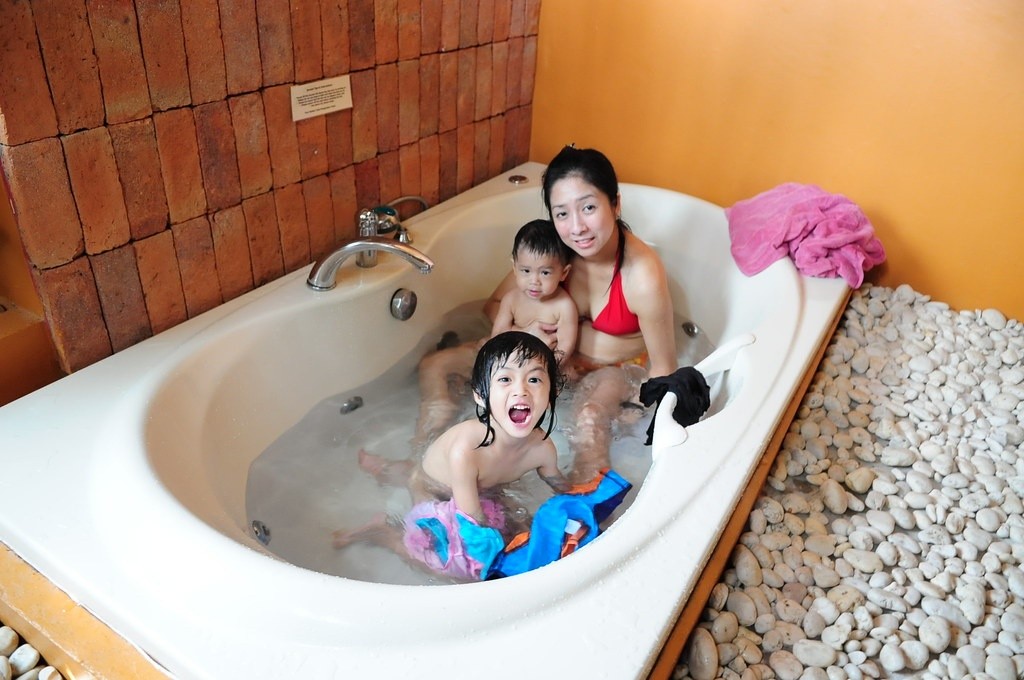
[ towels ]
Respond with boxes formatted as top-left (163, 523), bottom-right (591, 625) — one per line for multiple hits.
top-left (724, 182), bottom-right (886, 288)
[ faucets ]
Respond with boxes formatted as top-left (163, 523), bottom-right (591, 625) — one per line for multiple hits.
top-left (307, 237), bottom-right (435, 290)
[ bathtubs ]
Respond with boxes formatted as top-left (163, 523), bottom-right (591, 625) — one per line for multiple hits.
top-left (1, 161), bottom-right (853, 680)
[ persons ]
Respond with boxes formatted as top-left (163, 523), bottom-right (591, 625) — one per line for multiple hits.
top-left (475, 220), bottom-right (579, 385)
top-left (333, 330), bottom-right (574, 582)
top-left (411, 145), bottom-right (681, 484)
